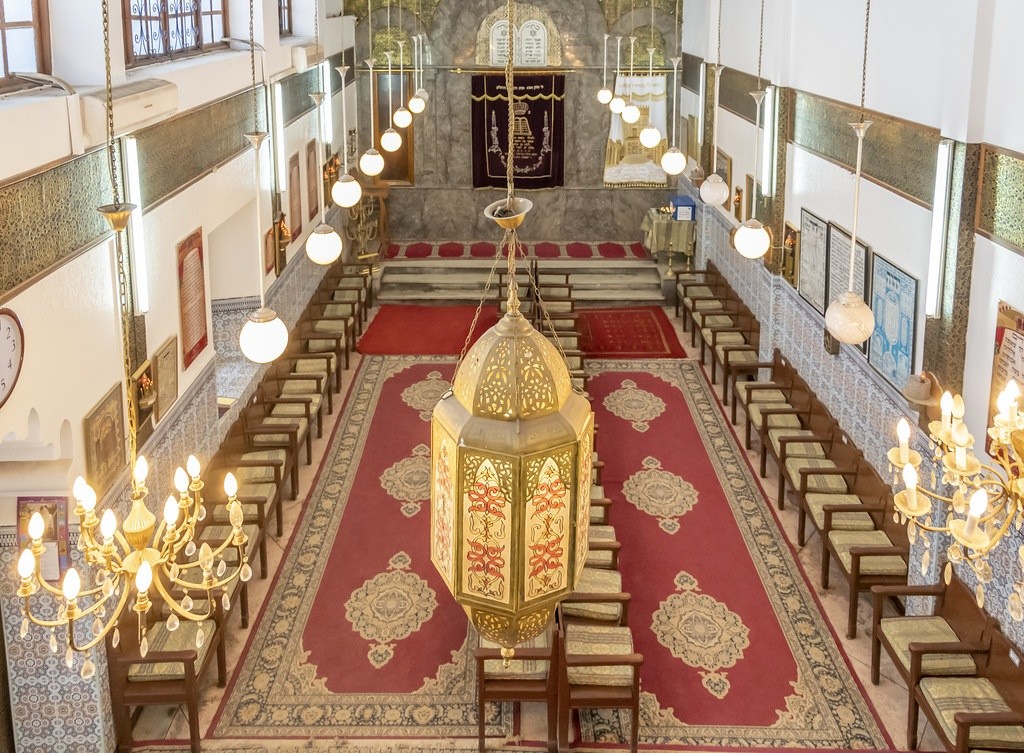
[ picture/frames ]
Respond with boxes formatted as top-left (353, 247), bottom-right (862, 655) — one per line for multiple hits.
top-left (130, 358), bottom-right (157, 433)
top-left (153, 333), bottom-right (179, 424)
top-left (680, 116), bottom-right (689, 164)
top-left (687, 113), bottom-right (698, 161)
top-left (349, 127), bottom-right (357, 156)
top-left (744, 172), bottom-right (763, 222)
top-left (781, 220), bottom-right (801, 289)
top-left (715, 146), bottom-right (732, 212)
top-left (732, 184), bottom-right (744, 223)
top-left (83, 380), bottom-right (127, 511)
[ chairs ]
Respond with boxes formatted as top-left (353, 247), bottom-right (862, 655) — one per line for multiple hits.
top-left (467, 256), bottom-right (647, 753)
top-left (670, 268), bottom-right (1024, 753)
top-left (102, 257), bottom-right (377, 753)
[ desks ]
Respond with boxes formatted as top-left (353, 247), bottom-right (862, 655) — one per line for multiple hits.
top-left (639, 208), bottom-right (696, 265)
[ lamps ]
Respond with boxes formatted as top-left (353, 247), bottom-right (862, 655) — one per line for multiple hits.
top-left (11, 0), bottom-right (430, 683)
top-left (597, 0), bottom-right (1024, 624)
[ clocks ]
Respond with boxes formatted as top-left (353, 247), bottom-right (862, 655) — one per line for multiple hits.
top-left (0, 306), bottom-right (25, 411)
